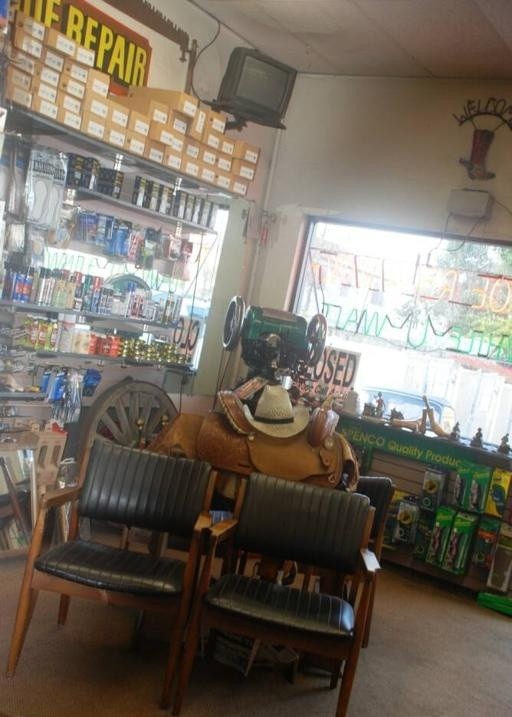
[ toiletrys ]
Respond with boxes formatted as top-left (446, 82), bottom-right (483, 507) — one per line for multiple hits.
top-left (0, 263), bottom-right (114, 315)
top-left (21, 316), bottom-right (59, 349)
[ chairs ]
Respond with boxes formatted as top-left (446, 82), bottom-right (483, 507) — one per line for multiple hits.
top-left (170, 471), bottom-right (382, 715)
top-left (5, 435), bottom-right (219, 714)
top-left (338, 473), bottom-right (397, 650)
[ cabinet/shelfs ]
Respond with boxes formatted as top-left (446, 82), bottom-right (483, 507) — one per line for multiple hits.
top-left (0, 133), bottom-right (220, 446)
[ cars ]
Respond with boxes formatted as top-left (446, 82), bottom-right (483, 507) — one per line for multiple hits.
top-left (359, 388), bottom-right (457, 435)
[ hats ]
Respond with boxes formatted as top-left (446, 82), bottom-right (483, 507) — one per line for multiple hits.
top-left (242, 383), bottom-right (311, 436)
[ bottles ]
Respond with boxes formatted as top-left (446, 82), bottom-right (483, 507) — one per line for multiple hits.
top-left (0, 259), bottom-right (184, 324)
top-left (23, 313), bottom-right (195, 365)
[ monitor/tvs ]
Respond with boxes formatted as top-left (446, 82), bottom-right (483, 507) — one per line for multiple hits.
top-left (218, 46), bottom-right (298, 123)
